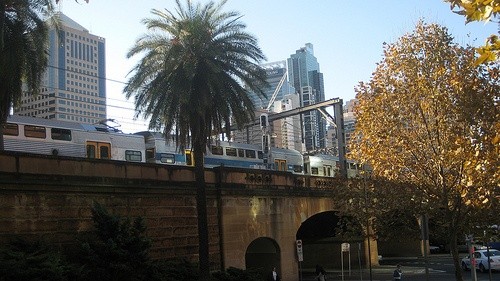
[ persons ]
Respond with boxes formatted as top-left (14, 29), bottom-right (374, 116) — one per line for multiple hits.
top-left (394, 264), bottom-right (402, 281)
top-left (313, 264), bottom-right (328, 281)
top-left (271, 266), bottom-right (278, 281)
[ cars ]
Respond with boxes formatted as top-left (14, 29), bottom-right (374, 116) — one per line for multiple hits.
top-left (461, 246), bottom-right (500, 273)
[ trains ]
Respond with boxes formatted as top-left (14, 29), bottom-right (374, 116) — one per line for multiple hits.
top-left (2, 114), bottom-right (374, 179)
